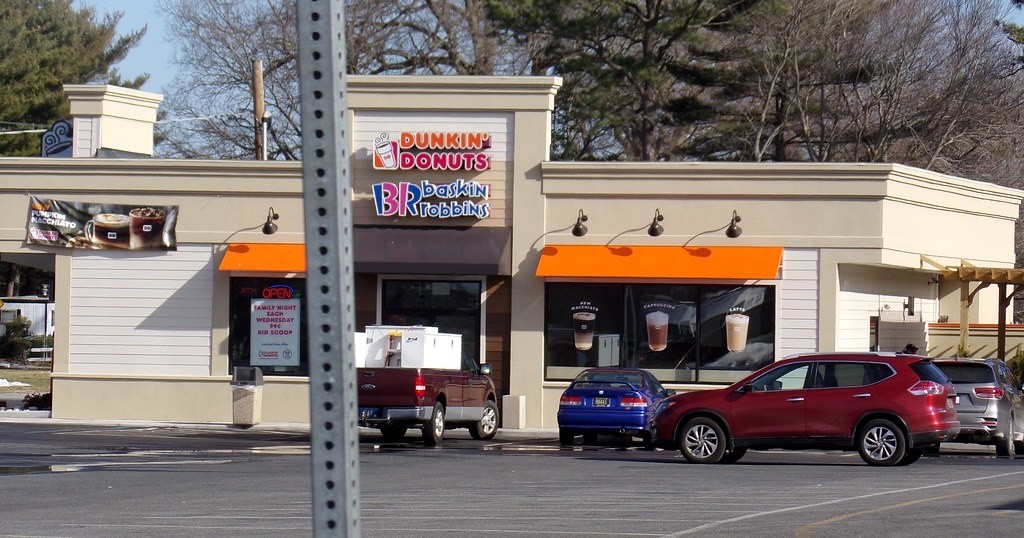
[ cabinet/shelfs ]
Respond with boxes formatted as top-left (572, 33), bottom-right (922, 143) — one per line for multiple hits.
top-left (364, 325), bottom-right (463, 369)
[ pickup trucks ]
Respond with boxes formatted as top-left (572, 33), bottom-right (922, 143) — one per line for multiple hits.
top-left (356, 343), bottom-right (502, 447)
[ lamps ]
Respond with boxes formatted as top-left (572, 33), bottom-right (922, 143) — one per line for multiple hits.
top-left (572, 209), bottom-right (588, 237)
top-left (648, 208), bottom-right (664, 237)
top-left (726, 209), bottom-right (742, 239)
top-left (262, 207), bottom-right (280, 234)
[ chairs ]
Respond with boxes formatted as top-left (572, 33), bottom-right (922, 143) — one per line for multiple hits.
top-left (816, 370), bottom-right (838, 388)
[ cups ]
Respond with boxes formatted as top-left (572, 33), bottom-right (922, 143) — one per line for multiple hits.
top-left (725, 314), bottom-right (749, 352)
top-left (573, 312), bottom-right (595, 351)
top-left (84, 213), bottom-right (130, 248)
top-left (129, 208), bottom-right (166, 249)
top-left (646, 312), bottom-right (669, 352)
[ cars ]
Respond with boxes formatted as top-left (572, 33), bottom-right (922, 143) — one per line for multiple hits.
top-left (932, 356), bottom-right (1023, 461)
top-left (647, 352), bottom-right (957, 465)
top-left (555, 365), bottom-right (675, 450)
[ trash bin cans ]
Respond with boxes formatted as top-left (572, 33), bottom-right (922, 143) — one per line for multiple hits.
top-left (230, 365), bottom-right (265, 424)
